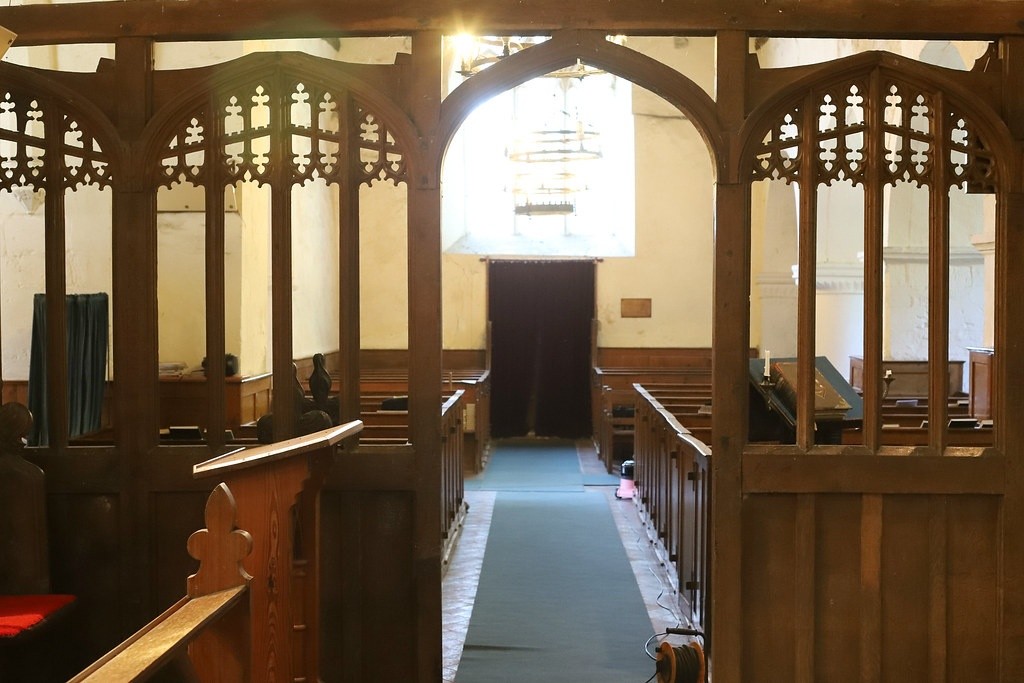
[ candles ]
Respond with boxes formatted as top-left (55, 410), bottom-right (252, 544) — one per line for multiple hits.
top-left (764, 350), bottom-right (771, 376)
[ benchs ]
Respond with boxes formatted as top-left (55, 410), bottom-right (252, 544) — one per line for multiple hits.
top-left (592, 367), bottom-right (993, 627)
top-left (240, 367), bottom-right (490, 575)
top-left (2, 595), bottom-right (78, 647)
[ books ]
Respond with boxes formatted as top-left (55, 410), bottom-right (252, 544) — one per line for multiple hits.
top-left (946, 399), bottom-right (993, 428)
top-left (159, 424), bottom-right (236, 439)
top-left (881, 399), bottom-right (928, 428)
top-left (769, 362), bottom-right (854, 421)
